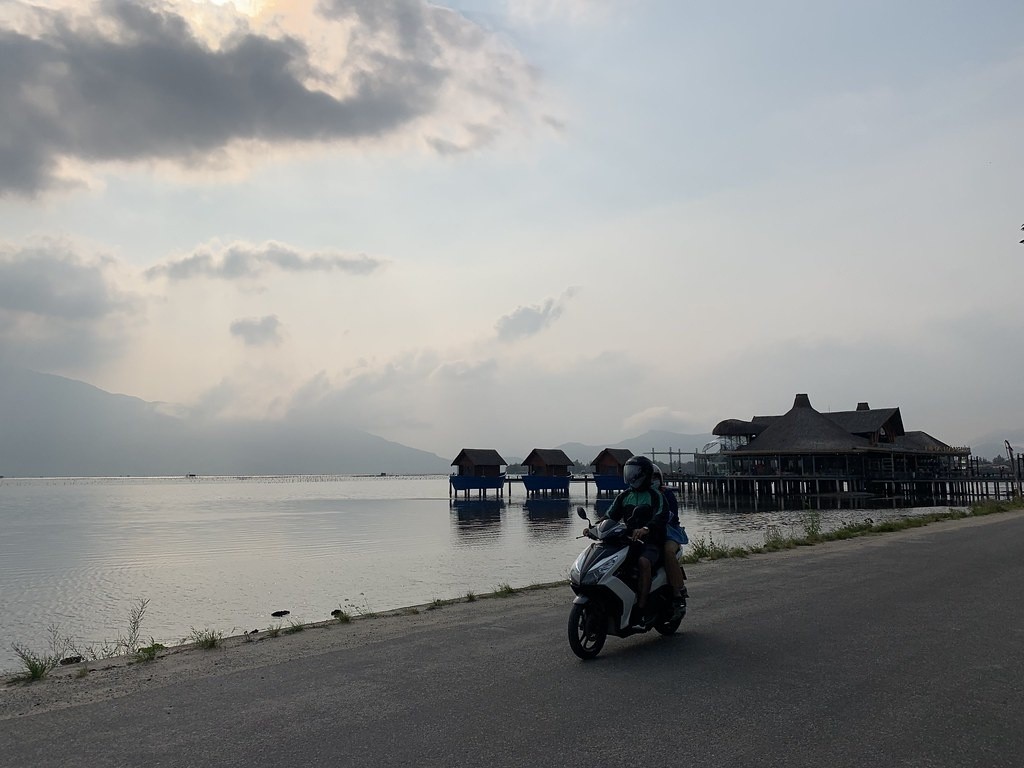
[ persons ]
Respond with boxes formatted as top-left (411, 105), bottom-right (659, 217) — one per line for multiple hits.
top-left (583, 456), bottom-right (688, 630)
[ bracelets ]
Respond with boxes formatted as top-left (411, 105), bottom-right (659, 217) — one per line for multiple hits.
top-left (643, 526), bottom-right (649, 533)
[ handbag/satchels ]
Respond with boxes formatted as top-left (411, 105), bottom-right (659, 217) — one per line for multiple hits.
top-left (666, 523), bottom-right (688, 544)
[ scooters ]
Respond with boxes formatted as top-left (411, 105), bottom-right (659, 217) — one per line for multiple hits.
top-left (568, 506), bottom-right (690, 659)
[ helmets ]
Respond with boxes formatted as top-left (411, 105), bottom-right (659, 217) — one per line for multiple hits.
top-left (653, 464), bottom-right (663, 485)
top-left (625, 456), bottom-right (653, 492)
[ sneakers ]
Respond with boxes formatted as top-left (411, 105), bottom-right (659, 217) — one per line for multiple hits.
top-left (632, 620), bottom-right (646, 630)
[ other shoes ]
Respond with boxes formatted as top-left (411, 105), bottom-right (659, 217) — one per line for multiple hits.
top-left (672, 596), bottom-right (686, 614)
